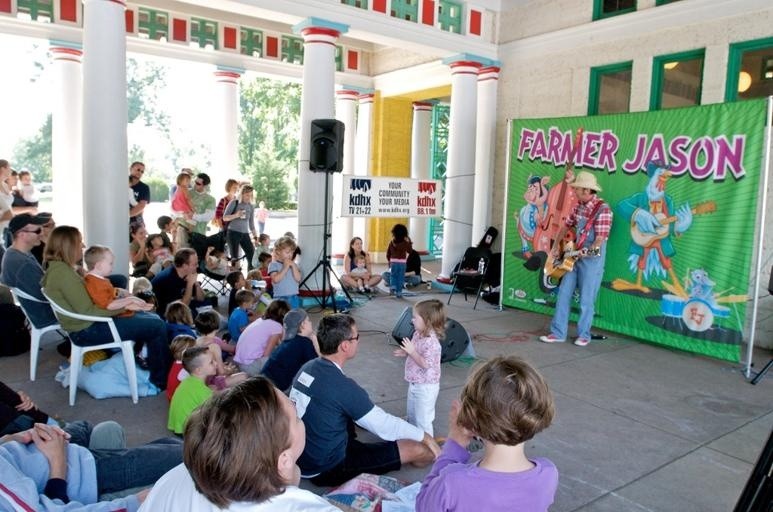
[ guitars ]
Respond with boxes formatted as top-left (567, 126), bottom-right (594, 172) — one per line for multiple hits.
top-left (632, 200), bottom-right (718, 247)
top-left (543, 241), bottom-right (602, 278)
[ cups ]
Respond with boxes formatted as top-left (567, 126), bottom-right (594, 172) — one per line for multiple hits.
top-left (239, 208), bottom-right (246, 219)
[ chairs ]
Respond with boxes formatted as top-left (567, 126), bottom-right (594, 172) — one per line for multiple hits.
top-left (8, 285), bottom-right (140, 409)
top-left (446, 243), bottom-right (493, 310)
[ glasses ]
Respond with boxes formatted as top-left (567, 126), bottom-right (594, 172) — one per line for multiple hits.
top-left (24, 227), bottom-right (41, 234)
top-left (137, 168), bottom-right (144, 173)
top-left (195, 180), bottom-right (202, 185)
top-left (349, 334), bottom-right (359, 341)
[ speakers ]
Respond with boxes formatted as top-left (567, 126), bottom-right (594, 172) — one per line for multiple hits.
top-left (391, 307), bottom-right (469, 363)
top-left (310, 119), bottom-right (345, 174)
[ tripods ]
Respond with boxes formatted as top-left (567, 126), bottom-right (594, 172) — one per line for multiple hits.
top-left (298, 173), bottom-right (352, 314)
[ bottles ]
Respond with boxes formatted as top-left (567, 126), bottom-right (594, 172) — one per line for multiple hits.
top-left (246, 279), bottom-right (266, 289)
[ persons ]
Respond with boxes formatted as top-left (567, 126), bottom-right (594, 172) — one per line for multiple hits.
top-left (339, 237), bottom-right (383, 294)
top-left (383, 224), bottom-right (423, 297)
top-left (0, 159), bottom-right (322, 435)
top-left (394, 299), bottom-right (445, 438)
top-left (0, 383), bottom-right (185, 512)
top-left (413, 353), bottom-right (560, 512)
top-left (138, 376), bottom-right (344, 512)
top-left (286, 314), bottom-right (441, 489)
top-left (537, 169), bottom-right (614, 346)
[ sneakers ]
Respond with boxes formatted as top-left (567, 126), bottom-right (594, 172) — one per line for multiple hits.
top-left (574, 338), bottom-right (588, 346)
top-left (540, 334), bottom-right (565, 343)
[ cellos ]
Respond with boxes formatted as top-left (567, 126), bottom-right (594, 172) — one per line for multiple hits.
top-left (534, 127), bottom-right (584, 253)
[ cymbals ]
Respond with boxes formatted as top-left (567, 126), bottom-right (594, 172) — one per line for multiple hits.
top-left (717, 295), bottom-right (750, 302)
top-left (660, 280), bottom-right (680, 296)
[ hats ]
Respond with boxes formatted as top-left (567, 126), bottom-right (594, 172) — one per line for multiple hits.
top-left (570, 173), bottom-right (602, 192)
top-left (9, 214), bottom-right (50, 233)
top-left (281, 308), bottom-right (307, 342)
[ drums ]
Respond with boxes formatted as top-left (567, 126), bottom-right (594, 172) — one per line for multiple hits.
top-left (661, 293), bottom-right (686, 318)
top-left (709, 304), bottom-right (733, 321)
